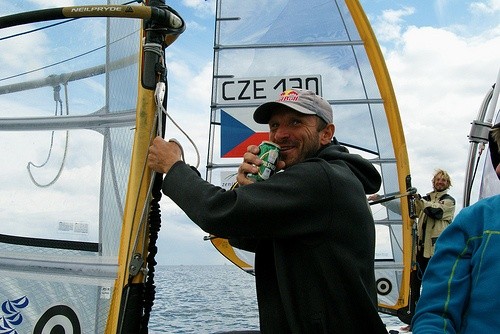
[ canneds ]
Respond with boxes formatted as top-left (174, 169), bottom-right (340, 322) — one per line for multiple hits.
top-left (246, 140), bottom-right (282, 182)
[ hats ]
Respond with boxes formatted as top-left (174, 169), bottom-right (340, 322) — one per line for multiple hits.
top-left (253, 88), bottom-right (333, 124)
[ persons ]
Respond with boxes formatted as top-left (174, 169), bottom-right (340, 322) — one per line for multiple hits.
top-left (368, 170), bottom-right (456, 332)
top-left (412, 121), bottom-right (500, 334)
top-left (146, 89), bottom-right (389, 334)
